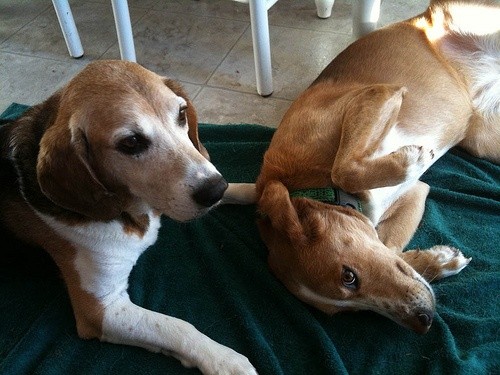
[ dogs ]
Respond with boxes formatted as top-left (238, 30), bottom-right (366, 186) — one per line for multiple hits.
top-left (255, 1), bottom-right (500, 334)
top-left (1, 59), bottom-right (258, 375)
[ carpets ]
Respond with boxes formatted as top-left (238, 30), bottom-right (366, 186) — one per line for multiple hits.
top-left (1, 101), bottom-right (500, 375)
